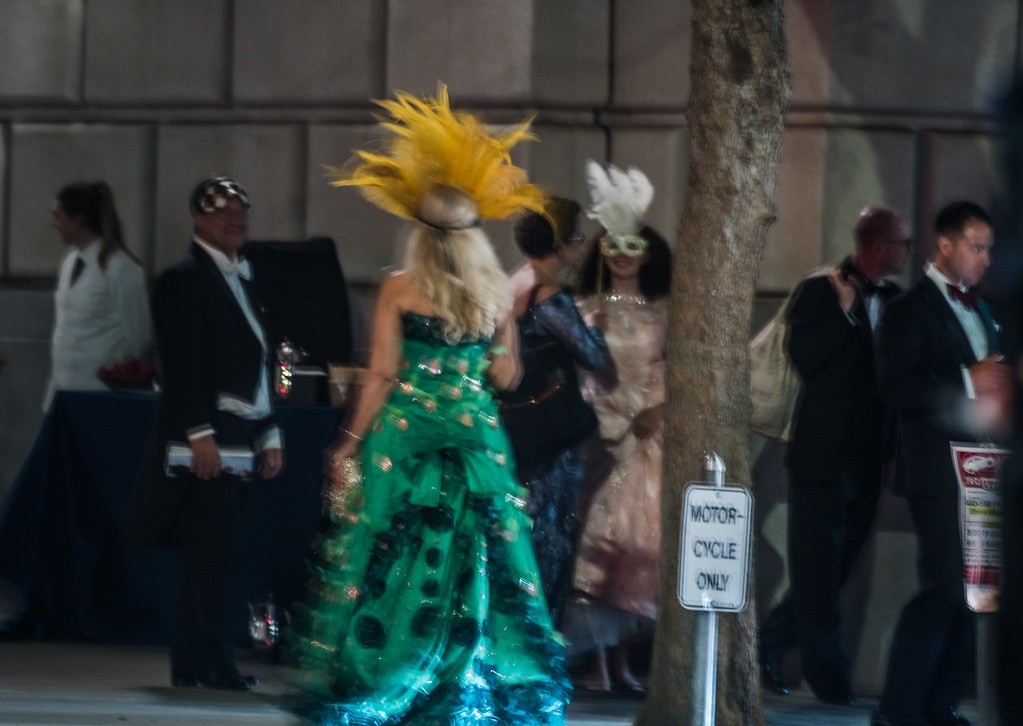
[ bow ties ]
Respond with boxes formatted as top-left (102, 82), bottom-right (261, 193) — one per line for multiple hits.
top-left (223, 256), bottom-right (252, 281)
top-left (864, 280), bottom-right (893, 298)
top-left (946, 283), bottom-right (970, 306)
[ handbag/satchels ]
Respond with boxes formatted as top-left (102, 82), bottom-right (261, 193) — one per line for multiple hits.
top-left (745, 269), bottom-right (832, 443)
top-left (500, 375), bottom-right (598, 469)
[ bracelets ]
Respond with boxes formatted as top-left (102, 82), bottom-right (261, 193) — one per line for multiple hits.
top-left (337, 426), bottom-right (365, 442)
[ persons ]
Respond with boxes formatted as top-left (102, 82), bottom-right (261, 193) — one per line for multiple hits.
top-left (147, 176), bottom-right (285, 691)
top-left (490, 196), bottom-right (620, 632)
top-left (570, 221), bottom-right (675, 699)
top-left (869, 200), bottom-right (1023, 726)
top-left (290, 181), bottom-right (576, 726)
top-left (756, 205), bottom-right (917, 708)
top-left (0, 179), bottom-right (151, 646)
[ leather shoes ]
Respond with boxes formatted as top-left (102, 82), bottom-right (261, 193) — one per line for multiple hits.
top-left (759, 632), bottom-right (790, 695)
top-left (871, 706), bottom-right (974, 726)
top-left (799, 643), bottom-right (856, 706)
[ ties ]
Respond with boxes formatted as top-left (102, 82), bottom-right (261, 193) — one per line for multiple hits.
top-left (70, 257), bottom-right (85, 284)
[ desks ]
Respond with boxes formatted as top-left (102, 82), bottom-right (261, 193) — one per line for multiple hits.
top-left (0, 390), bottom-right (344, 643)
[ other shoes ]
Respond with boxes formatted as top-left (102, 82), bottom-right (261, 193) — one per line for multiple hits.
top-left (171, 672), bottom-right (260, 691)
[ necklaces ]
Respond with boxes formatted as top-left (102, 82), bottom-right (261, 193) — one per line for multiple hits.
top-left (606, 292), bottom-right (649, 307)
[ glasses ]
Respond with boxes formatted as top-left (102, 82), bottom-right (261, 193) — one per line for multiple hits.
top-left (879, 237), bottom-right (912, 252)
top-left (568, 234), bottom-right (585, 245)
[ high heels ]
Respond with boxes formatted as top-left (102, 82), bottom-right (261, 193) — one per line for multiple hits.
top-left (614, 670), bottom-right (646, 694)
top-left (584, 652), bottom-right (611, 692)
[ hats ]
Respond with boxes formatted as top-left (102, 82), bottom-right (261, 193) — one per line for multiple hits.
top-left (189, 176), bottom-right (251, 213)
